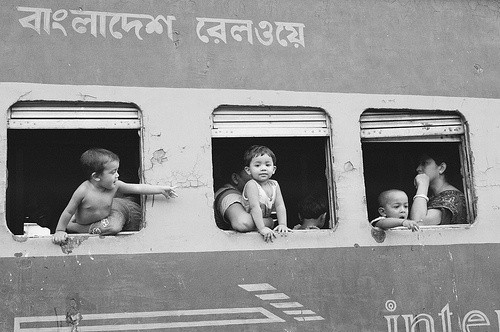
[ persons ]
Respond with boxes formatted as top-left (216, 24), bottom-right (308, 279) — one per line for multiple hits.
top-left (51, 148), bottom-right (178, 244)
top-left (410, 147), bottom-right (467, 225)
top-left (213, 145), bottom-right (293, 243)
top-left (370, 189), bottom-right (422, 231)
top-left (293, 195), bottom-right (328, 230)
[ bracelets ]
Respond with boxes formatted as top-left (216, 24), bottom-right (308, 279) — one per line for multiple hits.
top-left (413, 195), bottom-right (429, 202)
top-left (401, 220), bottom-right (405, 225)
